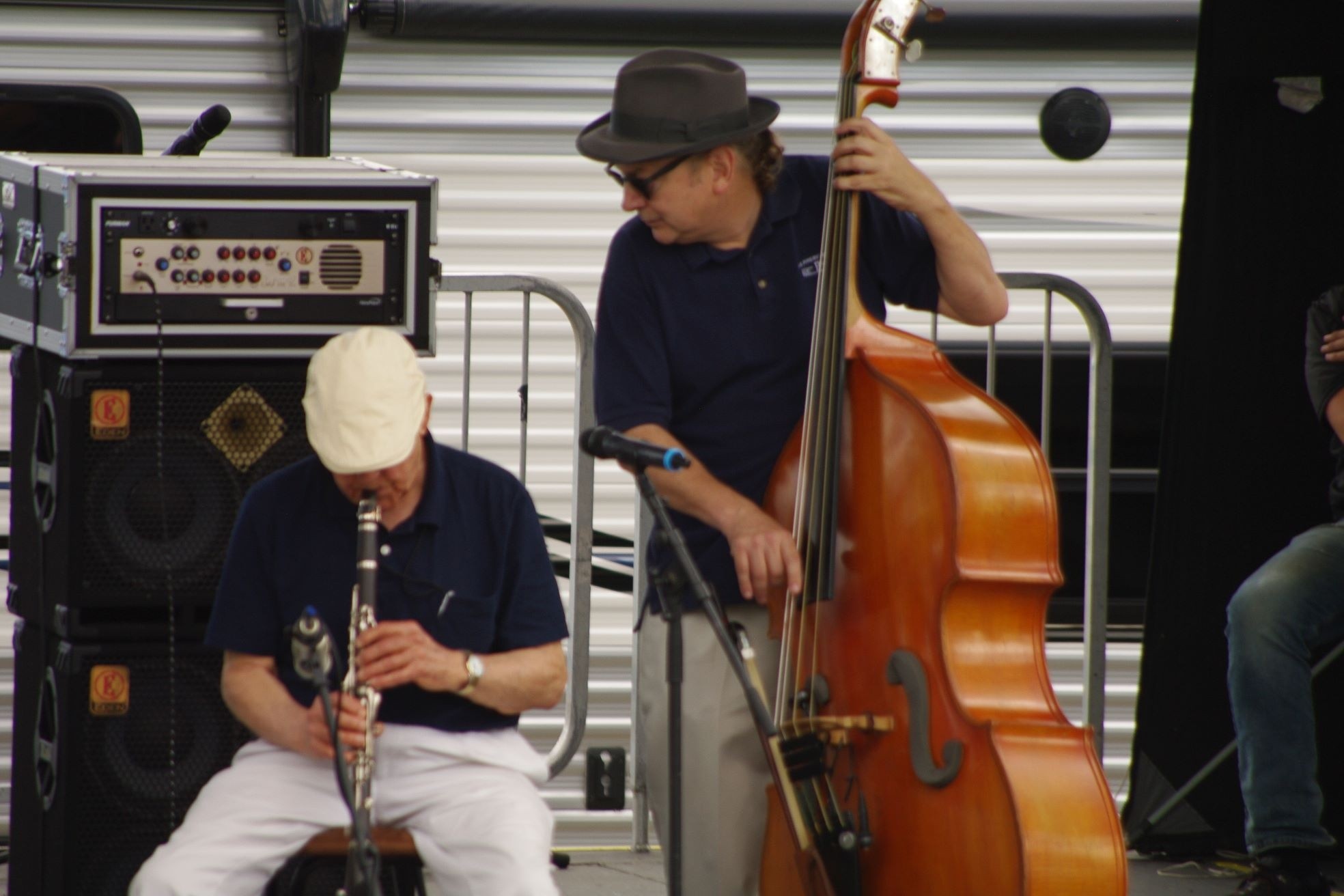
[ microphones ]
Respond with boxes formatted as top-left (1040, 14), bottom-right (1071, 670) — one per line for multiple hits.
top-left (290, 604), bottom-right (335, 680)
top-left (578, 425), bottom-right (692, 473)
top-left (161, 105), bottom-right (231, 156)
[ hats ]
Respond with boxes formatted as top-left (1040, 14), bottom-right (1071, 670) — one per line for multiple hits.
top-left (577, 48), bottom-right (782, 165)
top-left (303, 328), bottom-right (424, 474)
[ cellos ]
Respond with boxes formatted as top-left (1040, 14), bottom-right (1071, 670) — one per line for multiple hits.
top-left (759, 3), bottom-right (1128, 896)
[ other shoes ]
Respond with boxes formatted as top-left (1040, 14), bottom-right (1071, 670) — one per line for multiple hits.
top-left (1235, 848), bottom-right (1333, 896)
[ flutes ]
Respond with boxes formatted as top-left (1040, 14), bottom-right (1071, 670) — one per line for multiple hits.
top-left (343, 487), bottom-right (381, 896)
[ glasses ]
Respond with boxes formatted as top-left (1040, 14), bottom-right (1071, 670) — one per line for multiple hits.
top-left (604, 153), bottom-right (696, 196)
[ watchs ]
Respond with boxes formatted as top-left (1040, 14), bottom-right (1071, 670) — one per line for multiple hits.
top-left (456, 648), bottom-right (484, 698)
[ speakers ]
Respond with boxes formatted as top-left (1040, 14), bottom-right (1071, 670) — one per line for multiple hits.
top-left (5, 342), bottom-right (311, 641)
top-left (10, 622), bottom-right (304, 896)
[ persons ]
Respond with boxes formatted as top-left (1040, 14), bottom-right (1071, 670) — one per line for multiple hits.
top-left (125, 328), bottom-right (572, 896)
top-left (1223, 282), bottom-right (1344, 896)
top-left (577, 48), bottom-right (1010, 896)
